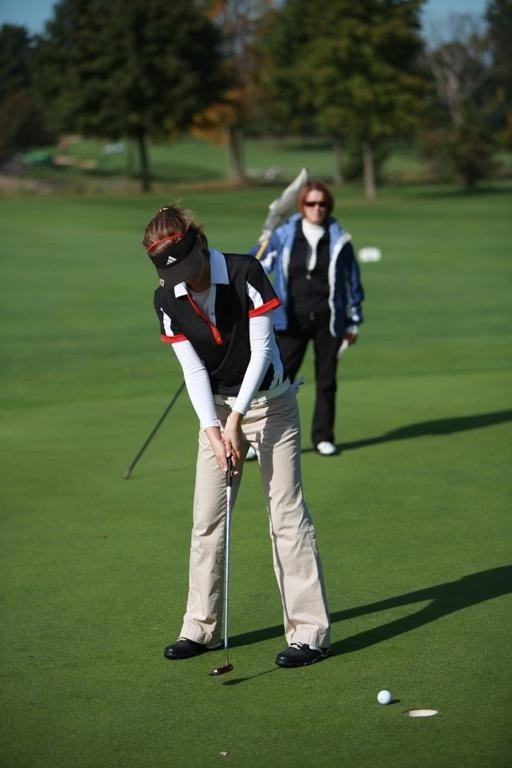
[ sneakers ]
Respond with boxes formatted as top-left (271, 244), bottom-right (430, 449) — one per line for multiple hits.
top-left (164, 640), bottom-right (207, 658)
top-left (317, 441), bottom-right (336, 455)
top-left (276, 644), bottom-right (332, 667)
top-left (245, 445), bottom-right (255, 458)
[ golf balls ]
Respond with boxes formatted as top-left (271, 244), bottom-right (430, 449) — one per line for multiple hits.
top-left (377, 690), bottom-right (391, 704)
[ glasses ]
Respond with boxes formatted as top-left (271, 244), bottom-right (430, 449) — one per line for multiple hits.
top-left (304, 202), bottom-right (327, 206)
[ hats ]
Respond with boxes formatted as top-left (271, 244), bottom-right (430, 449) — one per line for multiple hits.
top-left (148, 225), bottom-right (204, 287)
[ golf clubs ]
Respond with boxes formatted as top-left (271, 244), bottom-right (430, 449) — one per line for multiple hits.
top-left (123, 380), bottom-right (186, 479)
top-left (210, 455), bottom-right (234, 674)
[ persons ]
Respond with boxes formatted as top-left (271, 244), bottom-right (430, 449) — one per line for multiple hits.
top-left (241, 181), bottom-right (365, 465)
top-left (139, 202), bottom-right (335, 668)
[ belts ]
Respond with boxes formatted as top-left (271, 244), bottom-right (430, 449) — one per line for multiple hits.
top-left (214, 377), bottom-right (291, 406)
